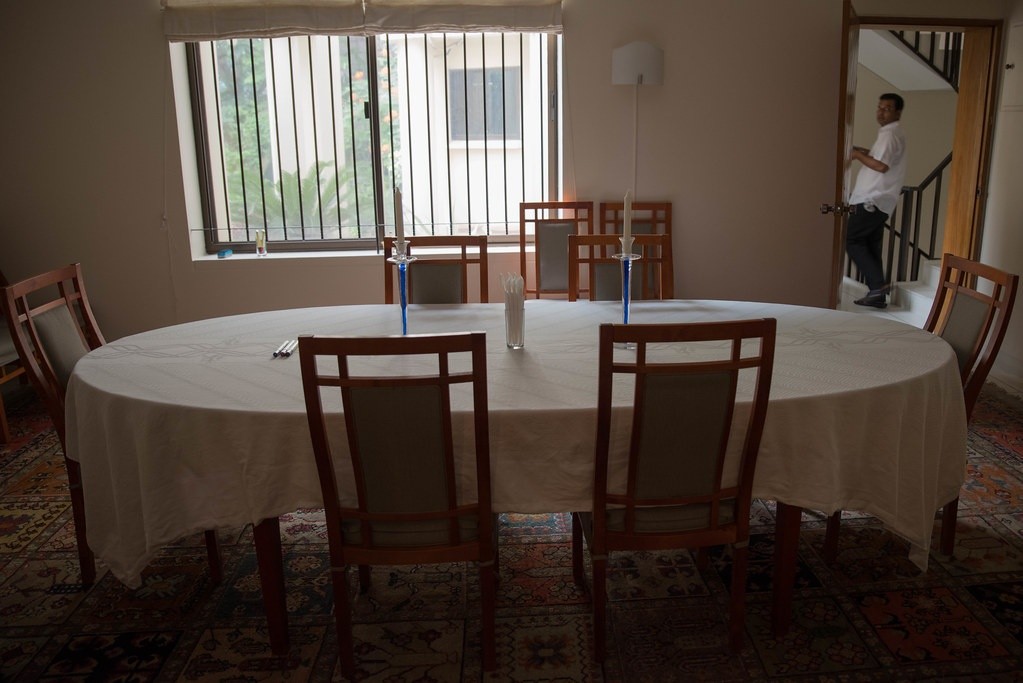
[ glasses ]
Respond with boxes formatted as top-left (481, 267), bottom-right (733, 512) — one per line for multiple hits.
top-left (875, 105), bottom-right (900, 113)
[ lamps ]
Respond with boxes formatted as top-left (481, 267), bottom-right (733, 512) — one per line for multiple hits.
top-left (609, 37), bottom-right (667, 219)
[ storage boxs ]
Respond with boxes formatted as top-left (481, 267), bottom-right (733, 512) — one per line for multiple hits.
top-left (217, 248), bottom-right (233, 258)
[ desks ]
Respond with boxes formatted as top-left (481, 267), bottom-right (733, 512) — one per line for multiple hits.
top-left (58, 301), bottom-right (977, 659)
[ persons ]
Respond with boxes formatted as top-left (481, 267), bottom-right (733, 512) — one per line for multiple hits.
top-left (847, 93), bottom-right (907, 309)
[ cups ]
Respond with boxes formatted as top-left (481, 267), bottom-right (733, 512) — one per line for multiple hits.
top-left (505, 309), bottom-right (525, 349)
top-left (255, 238), bottom-right (267, 256)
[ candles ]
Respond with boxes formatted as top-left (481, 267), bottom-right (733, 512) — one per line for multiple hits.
top-left (623, 188), bottom-right (633, 253)
top-left (392, 185), bottom-right (405, 244)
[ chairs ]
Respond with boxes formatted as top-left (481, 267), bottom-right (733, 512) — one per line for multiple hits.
top-left (294, 328), bottom-right (497, 677)
top-left (598, 199), bottom-right (675, 300)
top-left (568, 313), bottom-right (783, 658)
top-left (562, 232), bottom-right (677, 302)
top-left (517, 199), bottom-right (598, 301)
top-left (803, 252), bottom-right (1019, 568)
top-left (0, 261), bottom-right (223, 583)
top-left (384, 235), bottom-right (490, 307)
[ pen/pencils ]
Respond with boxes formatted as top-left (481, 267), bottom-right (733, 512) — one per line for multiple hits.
top-left (273, 340), bottom-right (289, 358)
top-left (287, 341), bottom-right (298, 355)
top-left (281, 340), bottom-right (295, 355)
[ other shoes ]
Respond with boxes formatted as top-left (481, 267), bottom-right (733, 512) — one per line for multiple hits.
top-left (853, 299), bottom-right (888, 309)
top-left (859, 284), bottom-right (891, 305)
